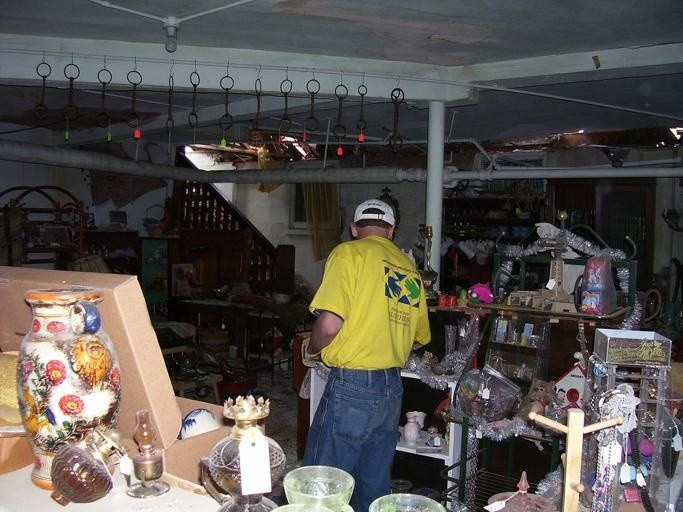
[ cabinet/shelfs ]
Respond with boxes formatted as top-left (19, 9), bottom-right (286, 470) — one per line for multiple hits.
top-left (425, 308), bottom-right (485, 354)
top-left (487, 308), bottom-right (600, 385)
top-left (585, 357), bottom-right (670, 472)
top-left (304, 355), bottom-right (482, 511)
top-left (442, 195), bottom-right (544, 299)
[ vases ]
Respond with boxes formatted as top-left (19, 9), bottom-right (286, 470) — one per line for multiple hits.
top-left (17, 286), bottom-right (122, 490)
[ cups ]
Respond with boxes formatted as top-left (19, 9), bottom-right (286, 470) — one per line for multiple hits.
top-left (404, 410), bottom-right (427, 429)
top-left (270, 465), bottom-right (357, 512)
top-left (367, 492), bottom-right (447, 512)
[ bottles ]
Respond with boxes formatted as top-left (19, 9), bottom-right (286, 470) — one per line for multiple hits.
top-left (403, 414), bottom-right (421, 446)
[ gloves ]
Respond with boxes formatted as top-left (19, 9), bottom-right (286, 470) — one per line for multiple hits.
top-left (301, 338), bottom-right (320, 368)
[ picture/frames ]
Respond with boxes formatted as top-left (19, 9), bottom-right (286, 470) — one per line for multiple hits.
top-left (171, 263), bottom-right (195, 300)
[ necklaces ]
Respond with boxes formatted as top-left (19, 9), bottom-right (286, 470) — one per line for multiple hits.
top-left (591, 427), bottom-right (614, 511)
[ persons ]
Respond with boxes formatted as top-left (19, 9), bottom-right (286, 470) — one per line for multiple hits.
top-left (299, 199), bottom-right (432, 511)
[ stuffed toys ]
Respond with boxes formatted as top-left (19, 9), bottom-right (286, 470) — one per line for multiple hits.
top-left (510, 376), bottom-right (559, 428)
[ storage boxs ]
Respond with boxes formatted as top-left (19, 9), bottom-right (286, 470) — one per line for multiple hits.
top-left (0, 265), bottom-right (266, 486)
top-left (592, 326), bottom-right (671, 368)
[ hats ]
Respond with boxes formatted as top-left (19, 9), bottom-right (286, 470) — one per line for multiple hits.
top-left (354, 198), bottom-right (395, 226)
